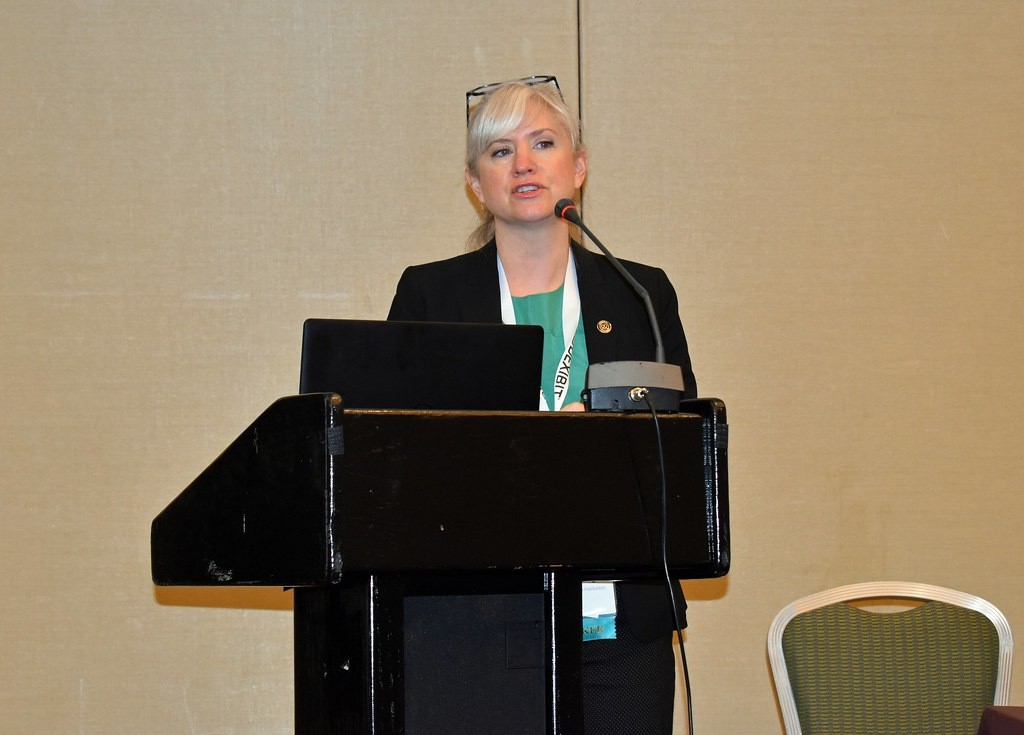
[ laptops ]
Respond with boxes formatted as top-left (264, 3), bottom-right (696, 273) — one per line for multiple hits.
top-left (299, 319), bottom-right (544, 413)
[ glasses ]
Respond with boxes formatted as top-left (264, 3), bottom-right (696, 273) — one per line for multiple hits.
top-left (465, 75), bottom-right (565, 129)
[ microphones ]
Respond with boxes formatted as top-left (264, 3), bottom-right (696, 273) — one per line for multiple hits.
top-left (554, 198), bottom-right (686, 412)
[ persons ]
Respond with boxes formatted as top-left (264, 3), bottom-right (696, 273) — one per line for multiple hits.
top-left (384, 74), bottom-right (698, 735)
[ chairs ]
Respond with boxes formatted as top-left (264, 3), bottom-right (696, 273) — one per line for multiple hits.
top-left (765, 580), bottom-right (1015, 735)
